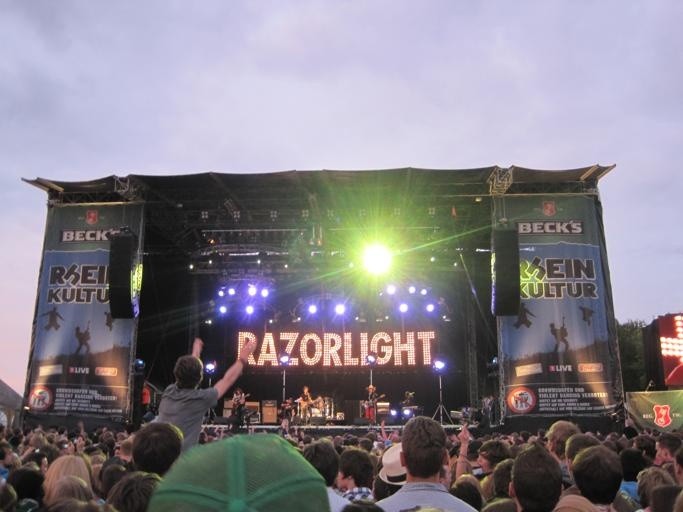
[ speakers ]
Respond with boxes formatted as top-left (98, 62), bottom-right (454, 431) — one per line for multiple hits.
top-left (310, 416), bottom-right (327, 426)
top-left (491, 228), bottom-right (520, 316)
top-left (214, 416), bottom-right (229, 424)
top-left (353, 417), bottom-right (370, 425)
top-left (108, 231), bottom-right (138, 320)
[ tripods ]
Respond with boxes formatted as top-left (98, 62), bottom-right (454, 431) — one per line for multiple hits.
top-left (205, 375), bottom-right (216, 424)
top-left (431, 372), bottom-right (455, 426)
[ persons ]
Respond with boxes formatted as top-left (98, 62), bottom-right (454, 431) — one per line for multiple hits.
top-left (104, 311), bottom-right (114, 331)
top-left (282, 395), bottom-right (294, 425)
top-left (362, 385), bottom-right (377, 422)
top-left (579, 305), bottom-right (594, 326)
top-left (294, 384), bottom-right (313, 425)
top-left (549, 323), bottom-right (569, 352)
top-left (142, 380), bottom-right (150, 416)
top-left (513, 303), bottom-right (536, 329)
top-left (0, 416), bottom-right (683, 512)
top-left (231, 388), bottom-right (248, 408)
top-left (39, 306), bottom-right (65, 330)
top-left (155, 336), bottom-right (255, 447)
top-left (75, 326), bottom-right (90, 354)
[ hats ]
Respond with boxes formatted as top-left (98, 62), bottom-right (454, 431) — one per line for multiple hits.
top-left (551, 494), bottom-right (600, 512)
top-left (451, 474), bottom-right (487, 507)
top-left (132, 422), bottom-right (341, 511)
top-left (543, 420), bottom-right (602, 460)
top-left (379, 442), bottom-right (407, 485)
top-left (0, 468), bottom-right (45, 512)
top-left (478, 439), bottom-right (513, 463)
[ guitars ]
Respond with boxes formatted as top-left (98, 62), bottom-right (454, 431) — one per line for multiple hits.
top-left (233, 394), bottom-right (250, 405)
top-left (363, 394), bottom-right (385, 409)
top-left (301, 398), bottom-right (321, 410)
